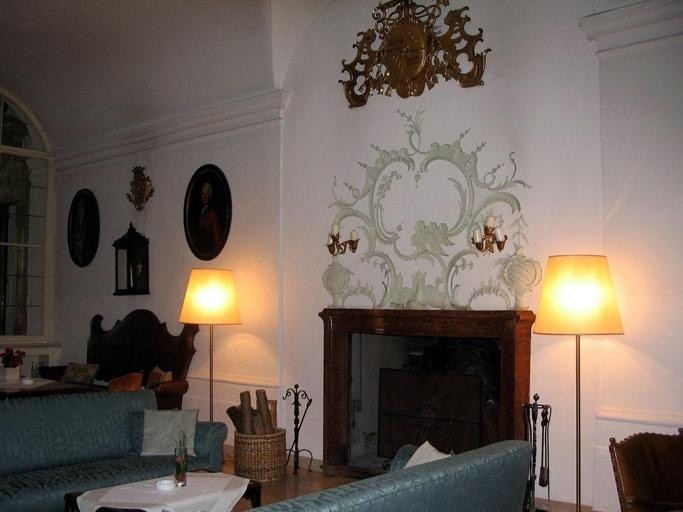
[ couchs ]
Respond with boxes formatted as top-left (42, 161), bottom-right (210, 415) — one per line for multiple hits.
top-left (39, 309), bottom-right (199, 410)
top-left (238, 440), bottom-right (535, 512)
top-left (0, 390), bottom-right (228, 512)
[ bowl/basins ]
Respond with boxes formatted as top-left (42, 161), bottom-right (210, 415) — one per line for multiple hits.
top-left (22, 377), bottom-right (34, 384)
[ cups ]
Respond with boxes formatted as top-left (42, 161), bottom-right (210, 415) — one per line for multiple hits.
top-left (21, 357), bottom-right (32, 376)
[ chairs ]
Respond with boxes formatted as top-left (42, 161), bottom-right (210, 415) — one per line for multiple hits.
top-left (608, 428), bottom-right (682, 512)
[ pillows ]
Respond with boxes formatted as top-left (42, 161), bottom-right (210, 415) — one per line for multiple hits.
top-left (403, 440), bottom-right (452, 469)
top-left (140, 408), bottom-right (199, 457)
top-left (127, 410), bottom-right (143, 457)
top-left (110, 372), bottom-right (143, 392)
top-left (60, 362), bottom-right (100, 386)
top-left (147, 366), bottom-right (173, 390)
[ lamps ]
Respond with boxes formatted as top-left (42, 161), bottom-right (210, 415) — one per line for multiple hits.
top-left (179, 268), bottom-right (243, 463)
top-left (472, 216), bottom-right (507, 252)
top-left (532, 255), bottom-right (625, 512)
top-left (327, 224), bottom-right (361, 255)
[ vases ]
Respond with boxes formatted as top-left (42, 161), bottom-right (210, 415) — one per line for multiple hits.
top-left (0, 367), bottom-right (20, 384)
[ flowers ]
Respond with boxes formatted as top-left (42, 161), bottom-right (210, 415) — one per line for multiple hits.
top-left (0, 347), bottom-right (26, 368)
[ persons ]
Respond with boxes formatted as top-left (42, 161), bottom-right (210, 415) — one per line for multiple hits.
top-left (72, 199), bottom-right (95, 261)
top-left (196, 183), bottom-right (223, 254)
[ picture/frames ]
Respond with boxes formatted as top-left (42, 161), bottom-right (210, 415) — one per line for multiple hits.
top-left (183, 164), bottom-right (232, 261)
top-left (68, 188), bottom-right (100, 267)
